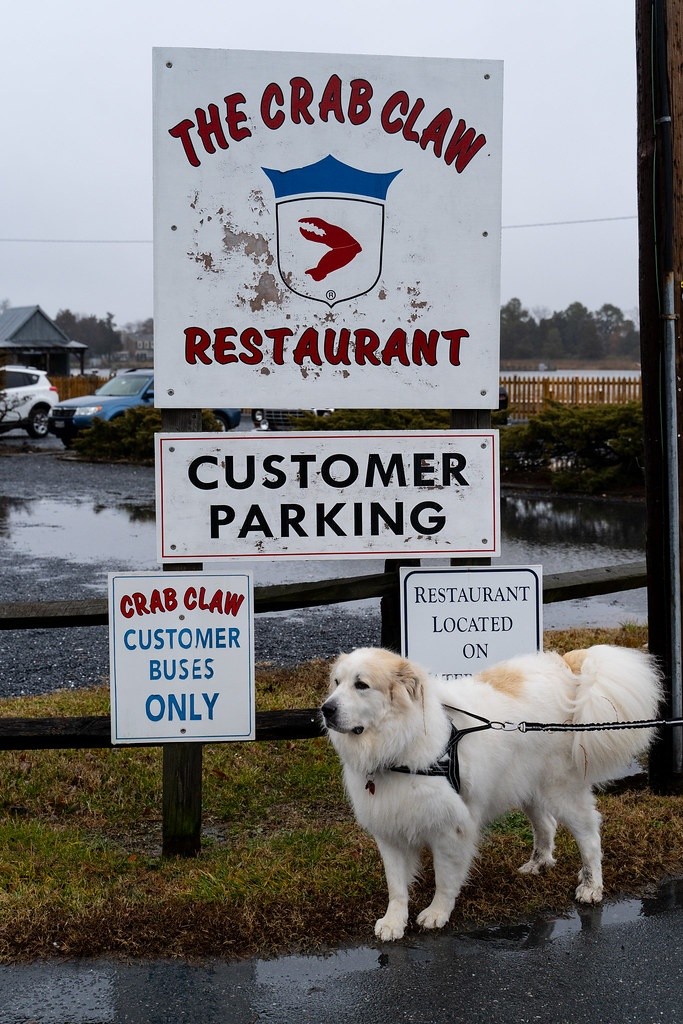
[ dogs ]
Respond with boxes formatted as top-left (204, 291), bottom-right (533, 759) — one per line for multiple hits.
top-left (316, 643), bottom-right (663, 943)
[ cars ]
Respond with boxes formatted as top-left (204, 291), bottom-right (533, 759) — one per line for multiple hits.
top-left (250, 388), bottom-right (509, 432)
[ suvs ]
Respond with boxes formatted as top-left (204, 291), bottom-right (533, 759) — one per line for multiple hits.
top-left (48, 365), bottom-right (241, 447)
top-left (0, 365), bottom-right (61, 439)
